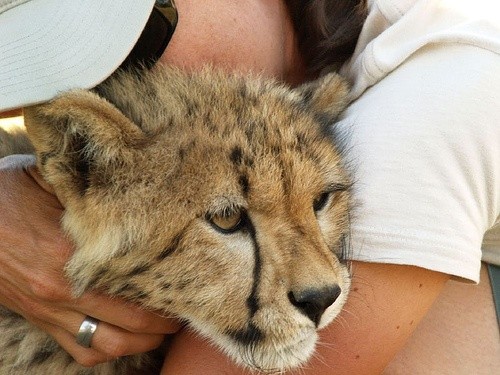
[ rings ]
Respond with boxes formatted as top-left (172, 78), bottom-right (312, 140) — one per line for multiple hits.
top-left (76, 316), bottom-right (100, 348)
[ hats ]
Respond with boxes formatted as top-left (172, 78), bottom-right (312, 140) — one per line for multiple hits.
top-left (1, 0), bottom-right (158, 119)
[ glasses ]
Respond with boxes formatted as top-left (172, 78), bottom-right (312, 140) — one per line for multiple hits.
top-left (117, 0), bottom-right (181, 76)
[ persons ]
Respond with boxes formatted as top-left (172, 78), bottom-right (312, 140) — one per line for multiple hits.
top-left (1, 1), bottom-right (499, 375)
